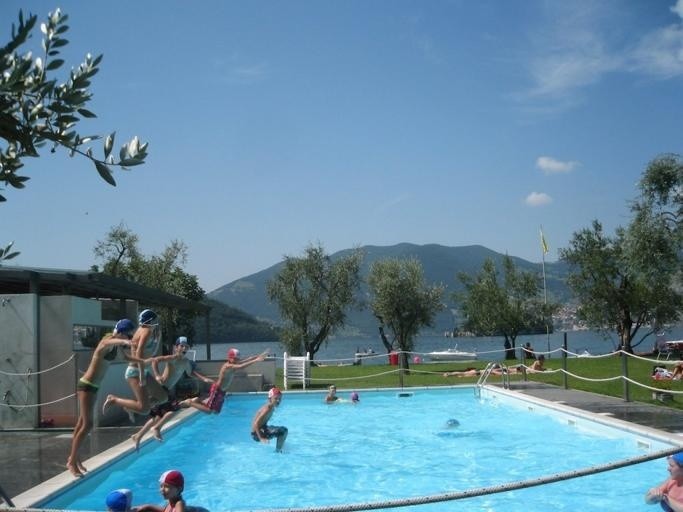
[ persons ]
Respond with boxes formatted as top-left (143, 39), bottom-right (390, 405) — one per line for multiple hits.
top-left (643, 453), bottom-right (683, 511)
top-left (102, 307), bottom-right (169, 418)
top-left (129, 336), bottom-right (217, 454)
top-left (250, 386), bottom-right (290, 454)
top-left (177, 347), bottom-right (266, 416)
top-left (131, 469), bottom-right (187, 512)
top-left (666, 351), bottom-right (683, 381)
top-left (350, 392), bottom-right (360, 403)
top-left (443, 341), bottom-right (548, 378)
top-left (65, 317), bottom-right (159, 477)
top-left (324, 383), bottom-right (343, 403)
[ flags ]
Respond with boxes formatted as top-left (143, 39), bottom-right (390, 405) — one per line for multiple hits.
top-left (540, 231), bottom-right (550, 255)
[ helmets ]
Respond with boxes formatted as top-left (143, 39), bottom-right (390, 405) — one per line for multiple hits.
top-left (138, 309), bottom-right (159, 326)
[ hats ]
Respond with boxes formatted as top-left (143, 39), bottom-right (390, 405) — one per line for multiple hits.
top-left (159, 470), bottom-right (184, 488)
top-left (268, 387), bottom-right (282, 398)
top-left (113, 318), bottom-right (136, 333)
top-left (228, 348), bottom-right (240, 359)
top-left (176, 336), bottom-right (191, 346)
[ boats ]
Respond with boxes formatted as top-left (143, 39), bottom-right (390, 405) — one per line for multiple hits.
top-left (424, 343), bottom-right (476, 362)
